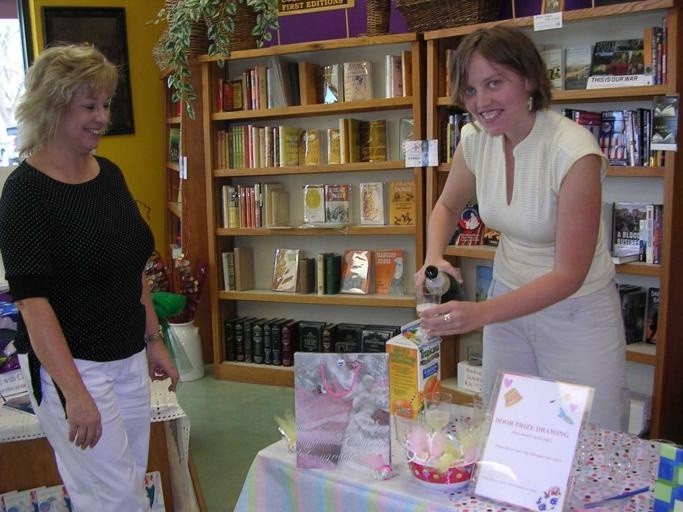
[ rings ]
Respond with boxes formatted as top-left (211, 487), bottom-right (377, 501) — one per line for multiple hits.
top-left (444, 314), bottom-right (450, 321)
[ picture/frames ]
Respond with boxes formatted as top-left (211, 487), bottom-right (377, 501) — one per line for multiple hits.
top-left (39, 6), bottom-right (136, 138)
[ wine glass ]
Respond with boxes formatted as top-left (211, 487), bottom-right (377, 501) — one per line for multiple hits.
top-left (416, 286), bottom-right (441, 340)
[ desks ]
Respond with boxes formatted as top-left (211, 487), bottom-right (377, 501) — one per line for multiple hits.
top-left (258, 404), bottom-right (658, 512)
top-left (0, 379), bottom-right (175, 511)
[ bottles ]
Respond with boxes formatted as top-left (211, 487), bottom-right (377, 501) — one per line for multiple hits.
top-left (424, 265), bottom-right (465, 303)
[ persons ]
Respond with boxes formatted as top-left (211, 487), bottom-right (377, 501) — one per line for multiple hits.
top-left (412, 24), bottom-right (628, 432)
top-left (0, 42), bottom-right (181, 512)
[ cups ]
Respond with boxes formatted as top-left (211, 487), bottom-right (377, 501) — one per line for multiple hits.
top-left (424, 392), bottom-right (489, 448)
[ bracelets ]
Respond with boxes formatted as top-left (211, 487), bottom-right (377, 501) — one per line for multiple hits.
top-left (143, 329), bottom-right (163, 344)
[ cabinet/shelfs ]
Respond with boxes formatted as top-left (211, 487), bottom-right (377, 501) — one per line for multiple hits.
top-left (196, 32), bottom-right (423, 386)
top-left (164, 58), bottom-right (205, 323)
top-left (423, 3), bottom-right (679, 438)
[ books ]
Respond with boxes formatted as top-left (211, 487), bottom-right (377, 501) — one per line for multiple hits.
top-left (539, 17), bottom-right (668, 92)
top-left (216, 117), bottom-right (414, 170)
top-left (440, 112), bottom-right (475, 164)
top-left (221, 246), bottom-right (253, 291)
top-left (214, 48), bottom-right (412, 112)
top-left (618, 284), bottom-right (660, 345)
top-left (221, 313), bottom-right (401, 367)
top-left (405, 139), bottom-right (438, 167)
top-left (445, 48), bottom-right (461, 98)
top-left (475, 266), bottom-right (493, 303)
top-left (221, 181), bottom-right (416, 229)
top-left (272, 248), bottom-right (406, 296)
top-left (561, 95), bottom-right (677, 167)
top-left (448, 203), bottom-right (501, 247)
top-left (602, 202), bottom-right (663, 264)
